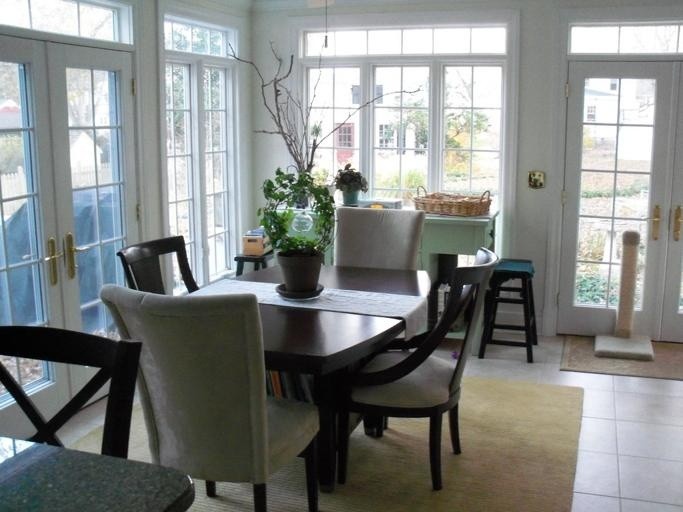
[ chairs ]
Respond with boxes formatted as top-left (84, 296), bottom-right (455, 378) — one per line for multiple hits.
top-left (0, 325), bottom-right (145, 463)
top-left (330, 202), bottom-right (420, 270)
top-left (117, 236), bottom-right (196, 299)
top-left (332, 246), bottom-right (498, 490)
top-left (101, 285), bottom-right (320, 510)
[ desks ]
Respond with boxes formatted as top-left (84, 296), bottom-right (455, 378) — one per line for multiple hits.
top-left (1, 437), bottom-right (196, 512)
top-left (418, 215), bottom-right (497, 331)
top-left (185, 263), bottom-right (442, 493)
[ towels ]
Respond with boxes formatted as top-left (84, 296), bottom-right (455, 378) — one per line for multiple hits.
top-left (189, 277), bottom-right (432, 341)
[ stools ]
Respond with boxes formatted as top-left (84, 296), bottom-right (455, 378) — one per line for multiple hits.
top-left (481, 257), bottom-right (540, 363)
top-left (233, 250), bottom-right (274, 275)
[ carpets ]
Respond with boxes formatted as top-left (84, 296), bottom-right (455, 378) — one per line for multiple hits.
top-left (559, 332), bottom-right (682, 386)
top-left (64, 374), bottom-right (585, 509)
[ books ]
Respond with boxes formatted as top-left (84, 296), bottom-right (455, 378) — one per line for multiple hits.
top-left (266, 369), bottom-right (314, 404)
top-left (245, 225), bottom-right (274, 248)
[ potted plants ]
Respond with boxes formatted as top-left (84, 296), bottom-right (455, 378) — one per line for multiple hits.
top-left (224, 40), bottom-right (418, 296)
top-left (335, 163), bottom-right (369, 205)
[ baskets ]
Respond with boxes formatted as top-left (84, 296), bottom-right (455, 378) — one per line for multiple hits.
top-left (411, 187), bottom-right (493, 217)
